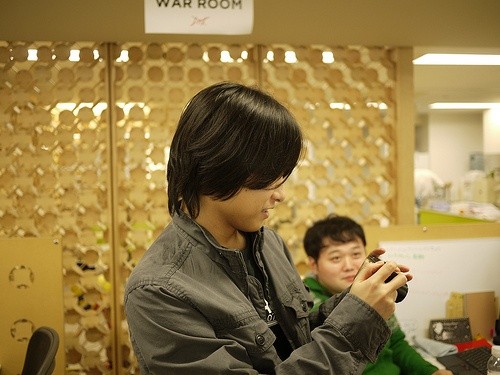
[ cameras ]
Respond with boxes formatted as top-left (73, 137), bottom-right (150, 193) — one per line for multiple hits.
top-left (352, 254), bottom-right (408, 304)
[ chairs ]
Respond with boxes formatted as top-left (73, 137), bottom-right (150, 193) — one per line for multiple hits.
top-left (20, 325), bottom-right (60, 375)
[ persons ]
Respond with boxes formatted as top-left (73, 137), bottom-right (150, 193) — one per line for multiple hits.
top-left (121, 80), bottom-right (415, 375)
top-left (301, 213), bottom-right (455, 374)
